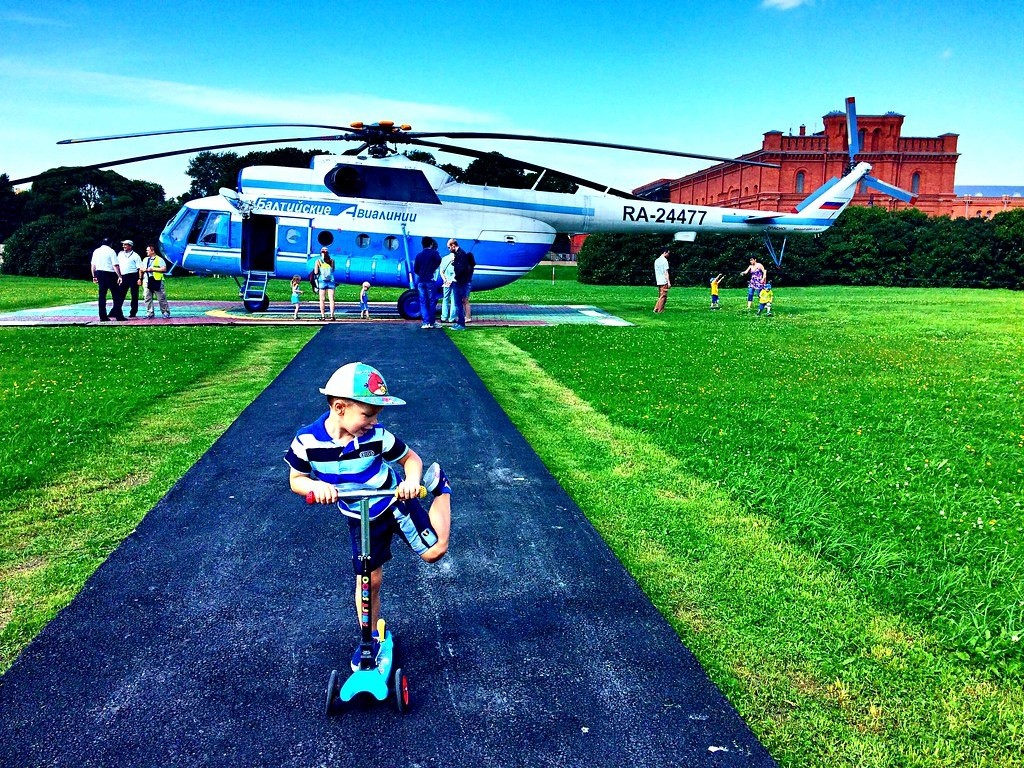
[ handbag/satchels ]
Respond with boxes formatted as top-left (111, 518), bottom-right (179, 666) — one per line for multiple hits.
top-left (148, 276), bottom-right (162, 292)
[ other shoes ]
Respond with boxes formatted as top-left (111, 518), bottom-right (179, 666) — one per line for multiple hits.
top-left (162, 311), bottom-right (170, 319)
top-left (143, 312), bottom-right (155, 319)
top-left (330, 317), bottom-right (335, 321)
top-left (319, 317), bottom-right (325, 321)
top-left (117, 315), bottom-right (128, 321)
top-left (767, 312), bottom-right (771, 316)
top-left (441, 319), bottom-right (448, 323)
top-left (449, 319), bottom-right (458, 323)
top-left (100, 316), bottom-right (110, 321)
top-left (465, 317), bottom-right (472, 322)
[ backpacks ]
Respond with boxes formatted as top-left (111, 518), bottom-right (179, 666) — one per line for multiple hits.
top-left (318, 259), bottom-right (333, 282)
top-left (466, 252), bottom-right (476, 283)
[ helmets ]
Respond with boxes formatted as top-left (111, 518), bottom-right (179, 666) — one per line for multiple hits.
top-left (102, 238), bottom-right (114, 245)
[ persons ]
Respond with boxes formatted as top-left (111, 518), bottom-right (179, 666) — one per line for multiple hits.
top-left (740, 255), bottom-right (773, 316)
top-left (710, 273), bottom-right (725, 309)
top-left (140, 244), bottom-right (171, 319)
top-left (414, 236), bottom-right (476, 331)
top-left (314, 247), bottom-right (336, 321)
top-left (291, 275), bottom-right (304, 319)
top-left (90, 238), bottom-right (142, 321)
top-left (282, 361), bottom-right (453, 673)
top-left (360, 281), bottom-right (373, 320)
top-left (653, 247), bottom-right (671, 314)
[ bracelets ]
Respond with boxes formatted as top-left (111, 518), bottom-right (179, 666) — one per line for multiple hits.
top-left (139, 277), bottom-right (142, 280)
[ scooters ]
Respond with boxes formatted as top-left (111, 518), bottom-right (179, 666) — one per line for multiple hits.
top-left (305, 486), bottom-right (428, 720)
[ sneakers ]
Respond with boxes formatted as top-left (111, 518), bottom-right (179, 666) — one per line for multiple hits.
top-left (433, 322), bottom-right (442, 328)
top-left (423, 462), bottom-right (448, 497)
top-left (351, 631), bottom-right (381, 672)
top-left (421, 324), bottom-right (434, 328)
top-left (449, 323), bottom-right (465, 331)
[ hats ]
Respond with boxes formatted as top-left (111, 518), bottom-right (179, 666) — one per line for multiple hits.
top-left (121, 240), bottom-right (133, 246)
top-left (319, 362), bottom-right (407, 406)
top-left (764, 284), bottom-right (771, 289)
top-left (321, 247), bottom-right (328, 252)
top-left (710, 278), bottom-right (714, 282)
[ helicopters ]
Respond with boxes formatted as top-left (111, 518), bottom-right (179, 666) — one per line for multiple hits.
top-left (10, 96), bottom-right (913, 324)
